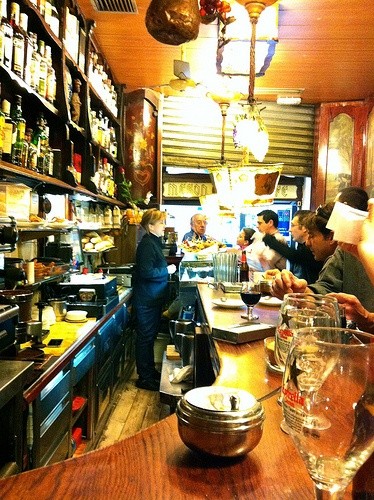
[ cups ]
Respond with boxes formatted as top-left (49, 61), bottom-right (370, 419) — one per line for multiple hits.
top-left (53, 301), bottom-right (67, 320)
top-left (263, 336), bottom-right (282, 372)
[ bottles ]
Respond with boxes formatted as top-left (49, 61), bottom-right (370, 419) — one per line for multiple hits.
top-left (238, 250), bottom-right (249, 283)
top-left (0, 0), bottom-right (127, 229)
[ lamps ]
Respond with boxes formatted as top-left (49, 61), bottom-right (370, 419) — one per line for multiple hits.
top-left (145, 0), bottom-right (304, 219)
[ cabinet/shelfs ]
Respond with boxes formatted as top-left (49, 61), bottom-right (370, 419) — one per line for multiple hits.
top-left (30, 305), bottom-right (133, 469)
top-left (0, 0), bottom-right (126, 283)
top-left (311, 100), bottom-right (374, 209)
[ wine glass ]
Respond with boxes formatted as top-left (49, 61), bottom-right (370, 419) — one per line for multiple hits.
top-left (277, 294), bottom-right (374, 500)
top-left (241, 282), bottom-right (262, 326)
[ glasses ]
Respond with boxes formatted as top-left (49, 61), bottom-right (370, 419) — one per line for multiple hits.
top-left (314, 205), bottom-right (330, 221)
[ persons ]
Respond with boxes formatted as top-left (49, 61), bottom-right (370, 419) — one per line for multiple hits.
top-left (181, 213), bottom-right (207, 255)
top-left (236, 227), bottom-right (257, 250)
top-left (250, 186), bottom-right (374, 345)
top-left (225, 210), bottom-right (287, 272)
top-left (132, 207), bottom-right (177, 391)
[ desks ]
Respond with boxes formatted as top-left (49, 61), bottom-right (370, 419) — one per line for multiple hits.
top-left (160, 351), bottom-right (192, 416)
top-left (0, 360), bottom-right (34, 408)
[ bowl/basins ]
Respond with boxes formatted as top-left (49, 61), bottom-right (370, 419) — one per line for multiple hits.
top-left (0, 290), bottom-right (33, 324)
top-left (175, 385), bottom-right (266, 461)
top-left (69, 309), bottom-right (89, 320)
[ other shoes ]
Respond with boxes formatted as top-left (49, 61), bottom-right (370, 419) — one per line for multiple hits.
top-left (135, 375), bottom-right (160, 392)
top-left (153, 371), bottom-right (161, 381)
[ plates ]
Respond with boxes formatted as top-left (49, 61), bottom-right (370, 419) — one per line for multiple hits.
top-left (259, 295), bottom-right (284, 306)
top-left (213, 298), bottom-right (245, 308)
